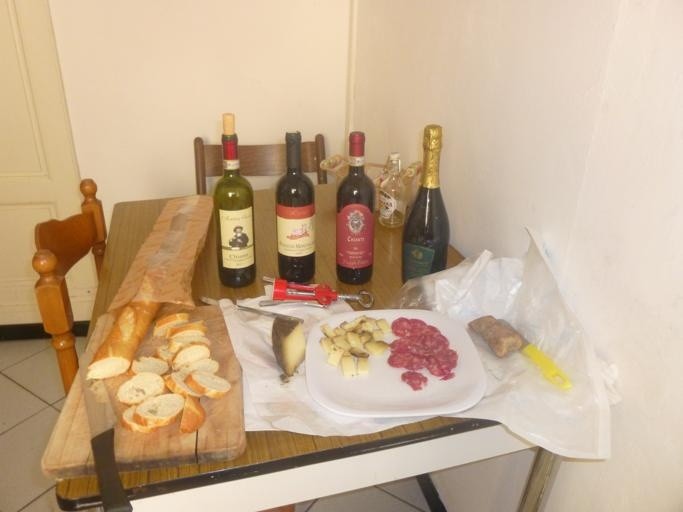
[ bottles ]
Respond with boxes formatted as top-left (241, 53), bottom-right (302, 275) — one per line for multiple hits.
top-left (332, 127), bottom-right (375, 288)
top-left (209, 113), bottom-right (258, 291)
top-left (401, 118), bottom-right (452, 288)
top-left (276, 132), bottom-right (317, 289)
top-left (380, 152), bottom-right (406, 227)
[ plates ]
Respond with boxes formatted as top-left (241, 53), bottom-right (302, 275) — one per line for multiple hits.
top-left (301, 308), bottom-right (482, 417)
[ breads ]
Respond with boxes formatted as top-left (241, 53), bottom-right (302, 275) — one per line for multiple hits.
top-left (87, 273), bottom-right (231, 434)
top-left (272, 316), bottom-right (306, 376)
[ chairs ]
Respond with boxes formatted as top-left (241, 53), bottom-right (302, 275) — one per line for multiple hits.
top-left (191, 132), bottom-right (329, 194)
top-left (31, 174), bottom-right (110, 397)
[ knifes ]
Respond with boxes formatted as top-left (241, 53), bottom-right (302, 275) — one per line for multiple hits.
top-left (496, 320), bottom-right (570, 389)
top-left (74, 361), bottom-right (136, 511)
top-left (199, 295), bottom-right (302, 323)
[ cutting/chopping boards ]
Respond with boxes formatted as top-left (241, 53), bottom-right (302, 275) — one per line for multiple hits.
top-left (40, 309), bottom-right (248, 477)
